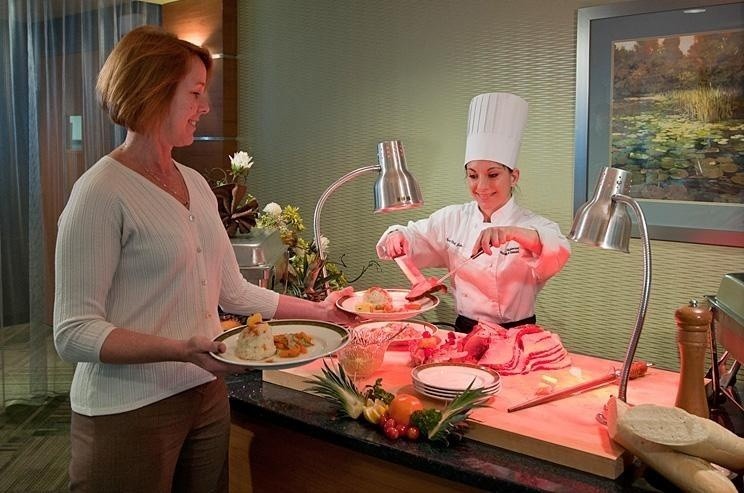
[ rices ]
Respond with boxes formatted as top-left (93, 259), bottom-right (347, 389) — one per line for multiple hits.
top-left (235, 326), bottom-right (276, 360)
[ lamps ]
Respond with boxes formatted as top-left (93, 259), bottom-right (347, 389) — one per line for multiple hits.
top-left (313, 139), bottom-right (425, 297)
top-left (566, 164), bottom-right (653, 428)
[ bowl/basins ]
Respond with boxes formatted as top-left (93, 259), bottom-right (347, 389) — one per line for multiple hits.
top-left (337, 328), bottom-right (393, 377)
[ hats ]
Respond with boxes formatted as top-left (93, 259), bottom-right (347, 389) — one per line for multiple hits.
top-left (464, 92), bottom-right (529, 171)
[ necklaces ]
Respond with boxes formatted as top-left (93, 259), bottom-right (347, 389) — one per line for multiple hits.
top-left (120, 143), bottom-right (190, 207)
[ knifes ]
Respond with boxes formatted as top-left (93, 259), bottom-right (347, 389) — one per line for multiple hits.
top-left (435, 244), bottom-right (494, 285)
top-left (393, 249), bottom-right (427, 287)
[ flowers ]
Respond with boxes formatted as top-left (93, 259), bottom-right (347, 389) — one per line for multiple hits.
top-left (217, 151), bottom-right (283, 232)
top-left (278, 205), bottom-right (351, 302)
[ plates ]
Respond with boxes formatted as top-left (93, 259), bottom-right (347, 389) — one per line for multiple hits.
top-left (354, 321), bottom-right (438, 345)
top-left (336, 288), bottom-right (438, 320)
top-left (209, 320), bottom-right (351, 369)
top-left (410, 363), bottom-right (501, 404)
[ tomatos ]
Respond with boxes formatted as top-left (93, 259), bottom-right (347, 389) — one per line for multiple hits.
top-left (379, 394), bottom-right (424, 439)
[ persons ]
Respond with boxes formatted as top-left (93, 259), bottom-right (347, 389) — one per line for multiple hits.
top-left (374, 156), bottom-right (573, 334)
top-left (51, 24), bottom-right (369, 491)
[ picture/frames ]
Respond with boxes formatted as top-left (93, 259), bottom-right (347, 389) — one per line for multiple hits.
top-left (573, 0), bottom-right (744, 250)
